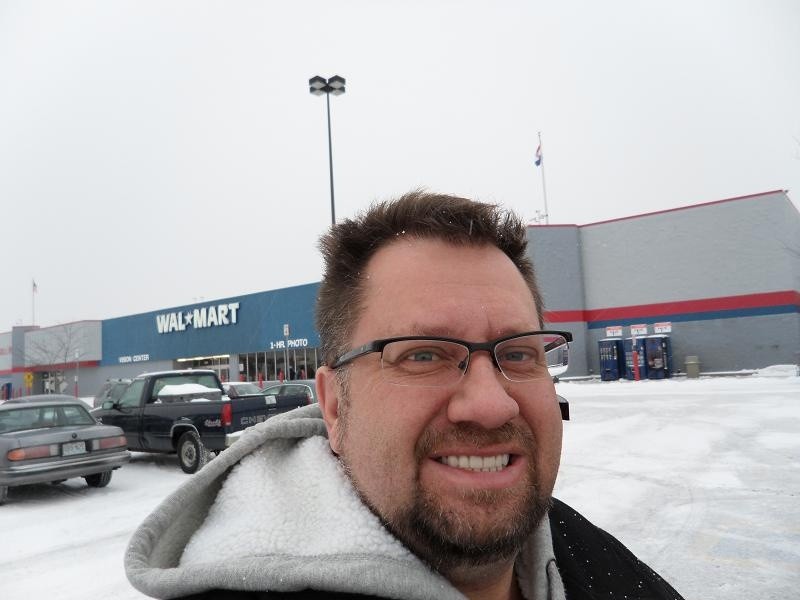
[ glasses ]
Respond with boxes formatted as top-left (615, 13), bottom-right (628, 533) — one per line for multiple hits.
top-left (330, 330), bottom-right (573, 389)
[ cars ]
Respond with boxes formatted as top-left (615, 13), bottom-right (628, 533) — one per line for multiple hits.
top-left (0, 393), bottom-right (132, 505)
top-left (220, 379), bottom-right (319, 405)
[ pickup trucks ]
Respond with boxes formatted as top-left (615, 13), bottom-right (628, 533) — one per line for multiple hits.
top-left (89, 369), bottom-right (312, 475)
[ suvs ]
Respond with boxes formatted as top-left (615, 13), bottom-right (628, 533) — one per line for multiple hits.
top-left (93, 377), bottom-right (133, 408)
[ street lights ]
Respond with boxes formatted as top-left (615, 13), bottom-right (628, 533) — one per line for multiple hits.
top-left (308, 74), bottom-right (347, 227)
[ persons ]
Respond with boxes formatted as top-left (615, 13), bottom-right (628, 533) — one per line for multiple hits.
top-left (125, 191), bottom-right (687, 600)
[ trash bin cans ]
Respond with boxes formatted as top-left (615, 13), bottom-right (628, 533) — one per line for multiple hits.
top-left (684, 356), bottom-right (700, 378)
